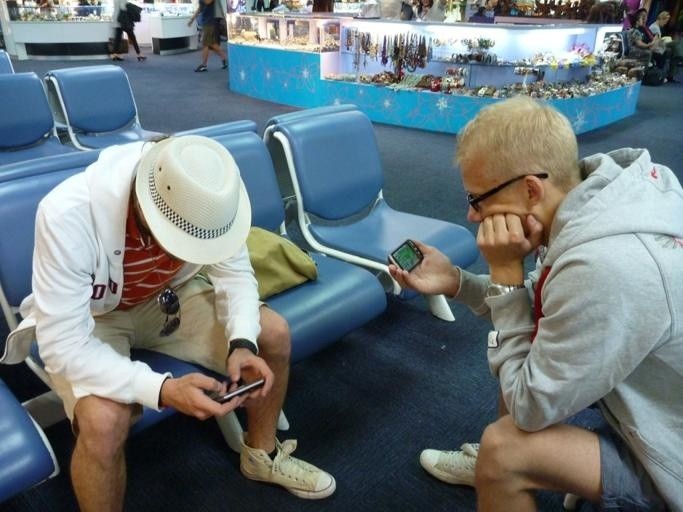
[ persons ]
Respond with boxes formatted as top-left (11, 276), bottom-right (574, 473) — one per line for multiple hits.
top-left (23, 0), bottom-right (33, 18)
top-left (648, 9), bottom-right (674, 82)
top-left (635, 7), bottom-right (673, 83)
top-left (35, 0), bottom-right (54, 14)
top-left (251, 0), bottom-right (278, 11)
top-left (311, 0), bottom-right (333, 12)
top-left (188, 0), bottom-right (229, 72)
top-left (400, 0), bottom-right (421, 21)
top-left (411, 0), bottom-right (447, 23)
top-left (74, 0), bottom-right (93, 17)
top-left (626, 13), bottom-right (669, 83)
top-left (388, 95), bottom-right (683, 512)
top-left (88, 0), bottom-right (102, 17)
top-left (0, 135), bottom-right (338, 512)
top-left (110, 0), bottom-right (147, 62)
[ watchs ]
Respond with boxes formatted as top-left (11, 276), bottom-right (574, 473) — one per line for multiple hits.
top-left (485, 280), bottom-right (527, 298)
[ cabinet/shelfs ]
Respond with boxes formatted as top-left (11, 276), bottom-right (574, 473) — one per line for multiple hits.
top-left (224, 11), bottom-right (645, 138)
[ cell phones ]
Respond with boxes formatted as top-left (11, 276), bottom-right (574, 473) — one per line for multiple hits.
top-left (217, 377), bottom-right (265, 402)
top-left (387, 238), bottom-right (424, 275)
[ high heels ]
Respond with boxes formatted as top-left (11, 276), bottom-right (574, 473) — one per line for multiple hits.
top-left (111, 54), bottom-right (124, 61)
top-left (137, 56), bottom-right (147, 61)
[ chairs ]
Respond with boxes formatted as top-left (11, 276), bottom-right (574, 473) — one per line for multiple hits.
top-left (0, 49), bottom-right (483, 512)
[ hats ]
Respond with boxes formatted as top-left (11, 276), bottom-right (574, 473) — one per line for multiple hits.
top-left (135, 134), bottom-right (252, 264)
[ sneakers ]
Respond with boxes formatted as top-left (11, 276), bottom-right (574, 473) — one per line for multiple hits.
top-left (194, 64), bottom-right (207, 71)
top-left (221, 59), bottom-right (226, 69)
top-left (418, 442), bottom-right (479, 487)
top-left (239, 435), bottom-right (336, 499)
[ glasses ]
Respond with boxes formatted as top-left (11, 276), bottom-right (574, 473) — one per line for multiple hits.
top-left (158, 286), bottom-right (180, 337)
top-left (465, 172), bottom-right (548, 212)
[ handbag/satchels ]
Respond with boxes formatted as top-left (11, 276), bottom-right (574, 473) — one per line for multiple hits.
top-left (643, 66), bottom-right (665, 86)
top-left (214, 18), bottom-right (227, 36)
top-left (118, 10), bottom-right (133, 29)
top-left (245, 226), bottom-right (317, 302)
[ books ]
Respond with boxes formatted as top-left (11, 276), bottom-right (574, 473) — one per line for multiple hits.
top-left (660, 34), bottom-right (674, 44)
